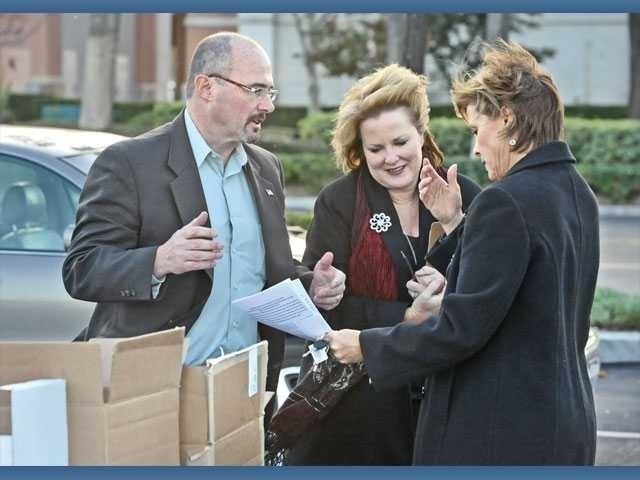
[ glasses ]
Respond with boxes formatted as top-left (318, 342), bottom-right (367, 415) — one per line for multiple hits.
top-left (208, 72), bottom-right (280, 102)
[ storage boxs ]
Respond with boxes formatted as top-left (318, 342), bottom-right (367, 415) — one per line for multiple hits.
top-left (1, 319), bottom-right (187, 465)
top-left (178, 338), bottom-right (277, 466)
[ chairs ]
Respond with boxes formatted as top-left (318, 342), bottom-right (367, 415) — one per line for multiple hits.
top-left (1, 177), bottom-right (67, 254)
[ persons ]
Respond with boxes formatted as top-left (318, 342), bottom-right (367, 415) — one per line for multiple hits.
top-left (61, 31), bottom-right (347, 434)
top-left (324, 37), bottom-right (600, 465)
top-left (263, 62), bottom-right (486, 466)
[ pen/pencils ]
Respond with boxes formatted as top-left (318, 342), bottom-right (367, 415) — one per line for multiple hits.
top-left (399, 249), bottom-right (418, 283)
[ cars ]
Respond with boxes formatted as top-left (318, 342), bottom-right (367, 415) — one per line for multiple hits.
top-left (0, 123), bottom-right (311, 366)
